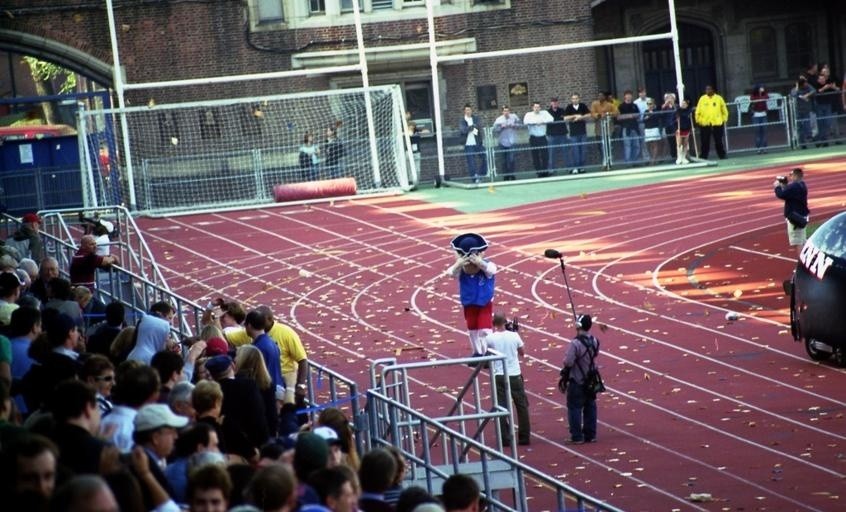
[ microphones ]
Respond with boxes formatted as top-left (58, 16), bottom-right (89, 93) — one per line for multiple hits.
top-left (544, 249), bottom-right (562, 259)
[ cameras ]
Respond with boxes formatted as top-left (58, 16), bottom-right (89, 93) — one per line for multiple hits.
top-left (776, 176), bottom-right (788, 184)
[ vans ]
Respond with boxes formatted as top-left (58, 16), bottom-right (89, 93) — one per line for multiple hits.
top-left (0, 123), bottom-right (113, 179)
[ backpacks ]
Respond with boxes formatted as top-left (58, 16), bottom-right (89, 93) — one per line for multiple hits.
top-left (571, 334), bottom-right (605, 396)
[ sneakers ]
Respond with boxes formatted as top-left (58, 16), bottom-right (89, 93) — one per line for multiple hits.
top-left (502, 436), bottom-right (597, 449)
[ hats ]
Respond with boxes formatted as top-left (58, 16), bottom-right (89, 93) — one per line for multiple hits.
top-left (21, 213), bottom-right (43, 224)
top-left (204, 336), bottom-right (233, 377)
top-left (311, 426), bottom-right (342, 447)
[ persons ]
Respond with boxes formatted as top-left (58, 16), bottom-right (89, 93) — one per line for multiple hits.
top-left (563, 92), bottom-right (591, 174)
top-left (751, 62), bottom-right (846, 153)
top-left (547, 98), bottom-right (573, 174)
top-left (592, 80), bottom-right (729, 159)
top-left (298, 134), bottom-right (322, 182)
top-left (523, 101), bottom-right (555, 178)
top-left (403, 122), bottom-right (422, 191)
top-left (492, 104), bottom-right (522, 180)
top-left (773, 168), bottom-right (810, 255)
top-left (459, 103), bottom-right (488, 182)
top-left (325, 126), bottom-right (347, 179)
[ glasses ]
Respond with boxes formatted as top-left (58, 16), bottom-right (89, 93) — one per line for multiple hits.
top-left (92, 375), bottom-right (115, 382)
